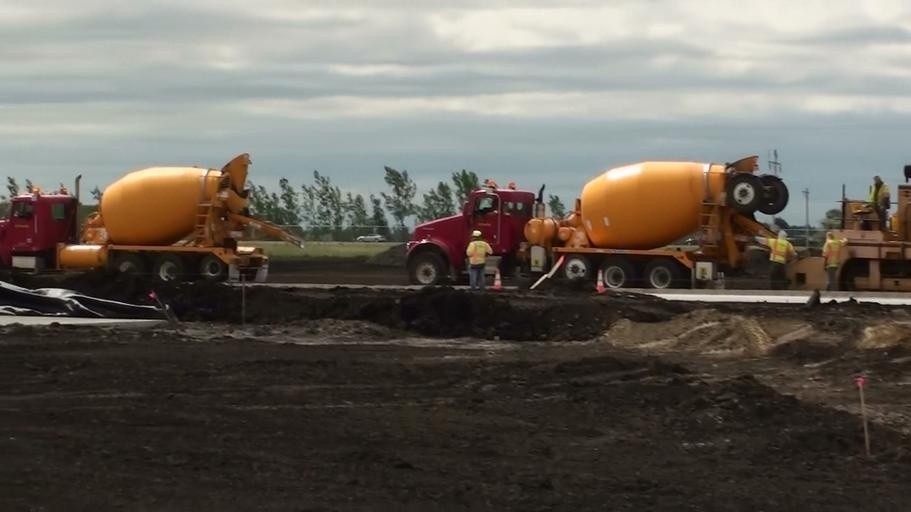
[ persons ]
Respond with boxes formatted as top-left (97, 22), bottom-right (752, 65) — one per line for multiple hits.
top-left (869, 175), bottom-right (890, 228)
top-left (466, 230), bottom-right (494, 292)
top-left (755, 230), bottom-right (797, 289)
top-left (822, 231), bottom-right (848, 290)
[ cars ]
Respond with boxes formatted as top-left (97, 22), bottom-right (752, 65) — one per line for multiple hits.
top-left (357, 234), bottom-right (387, 241)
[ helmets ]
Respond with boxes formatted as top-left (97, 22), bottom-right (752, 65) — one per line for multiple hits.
top-left (472, 231), bottom-right (482, 237)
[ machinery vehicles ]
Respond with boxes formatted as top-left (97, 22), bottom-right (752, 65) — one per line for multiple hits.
top-left (405, 154), bottom-right (789, 289)
top-left (0, 152), bottom-right (304, 283)
